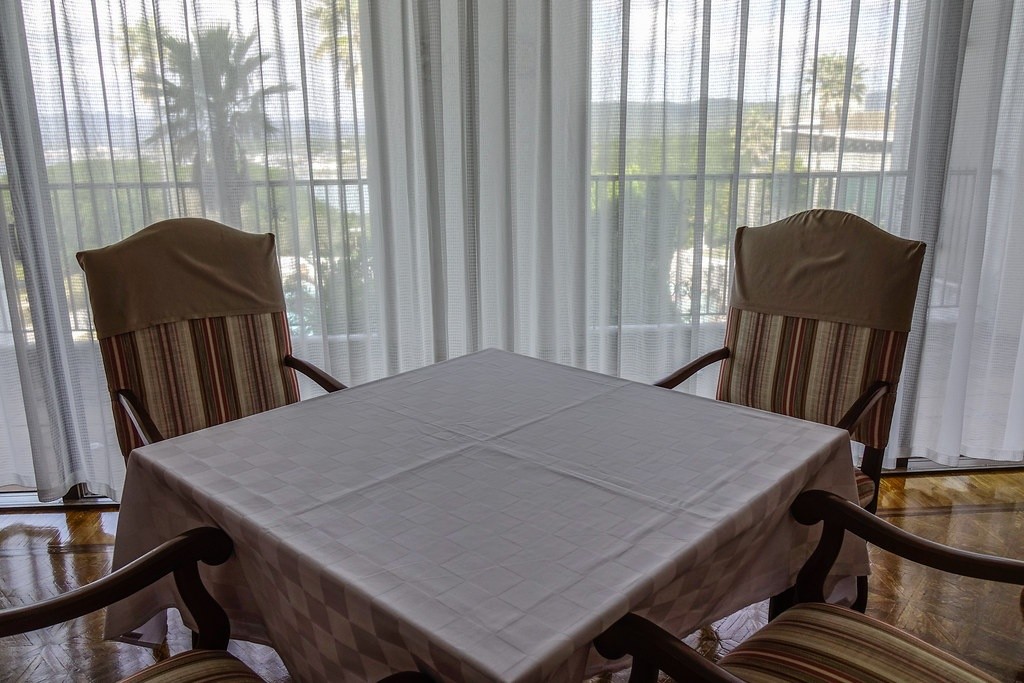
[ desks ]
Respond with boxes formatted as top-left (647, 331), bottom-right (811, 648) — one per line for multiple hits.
top-left (98, 348), bottom-right (871, 683)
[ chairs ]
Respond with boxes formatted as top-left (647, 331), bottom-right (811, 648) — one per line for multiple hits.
top-left (75, 213), bottom-right (347, 650)
top-left (0, 525), bottom-right (431, 683)
top-left (595, 488), bottom-right (1024, 683)
top-left (587, 207), bottom-right (928, 683)
